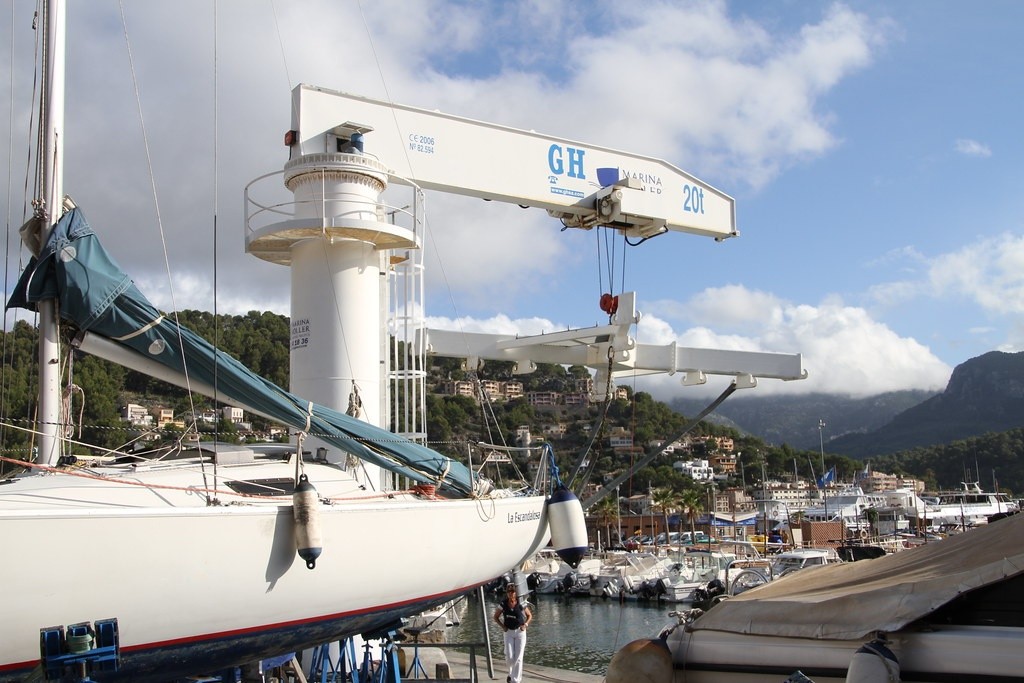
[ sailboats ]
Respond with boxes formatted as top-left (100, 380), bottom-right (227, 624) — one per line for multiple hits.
top-left (0, 0), bottom-right (806, 683)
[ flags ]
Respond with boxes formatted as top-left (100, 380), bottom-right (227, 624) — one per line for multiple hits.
top-left (817, 467), bottom-right (834, 488)
top-left (860, 464), bottom-right (868, 482)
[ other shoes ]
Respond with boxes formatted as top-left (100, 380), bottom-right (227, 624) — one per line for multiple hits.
top-left (506, 676), bottom-right (511, 683)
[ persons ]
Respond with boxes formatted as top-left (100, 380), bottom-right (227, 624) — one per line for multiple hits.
top-left (494, 585), bottom-right (532, 682)
top-left (595, 541), bottom-right (607, 559)
top-left (628, 539), bottom-right (640, 552)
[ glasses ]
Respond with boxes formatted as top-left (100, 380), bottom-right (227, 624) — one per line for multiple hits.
top-left (507, 590), bottom-right (515, 593)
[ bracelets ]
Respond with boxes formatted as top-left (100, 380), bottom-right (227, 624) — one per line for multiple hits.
top-left (525, 623), bottom-right (529, 627)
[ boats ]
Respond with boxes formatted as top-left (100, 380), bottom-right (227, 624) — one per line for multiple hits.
top-left (528, 471), bottom-right (1023, 605)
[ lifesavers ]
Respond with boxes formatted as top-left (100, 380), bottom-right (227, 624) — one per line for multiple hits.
top-left (890, 503), bottom-right (895, 507)
top-left (897, 503), bottom-right (901, 507)
top-left (773, 510), bottom-right (778, 516)
top-left (860, 529), bottom-right (868, 539)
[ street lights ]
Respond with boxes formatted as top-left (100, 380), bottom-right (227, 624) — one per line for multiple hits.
top-left (817, 418), bottom-right (828, 522)
